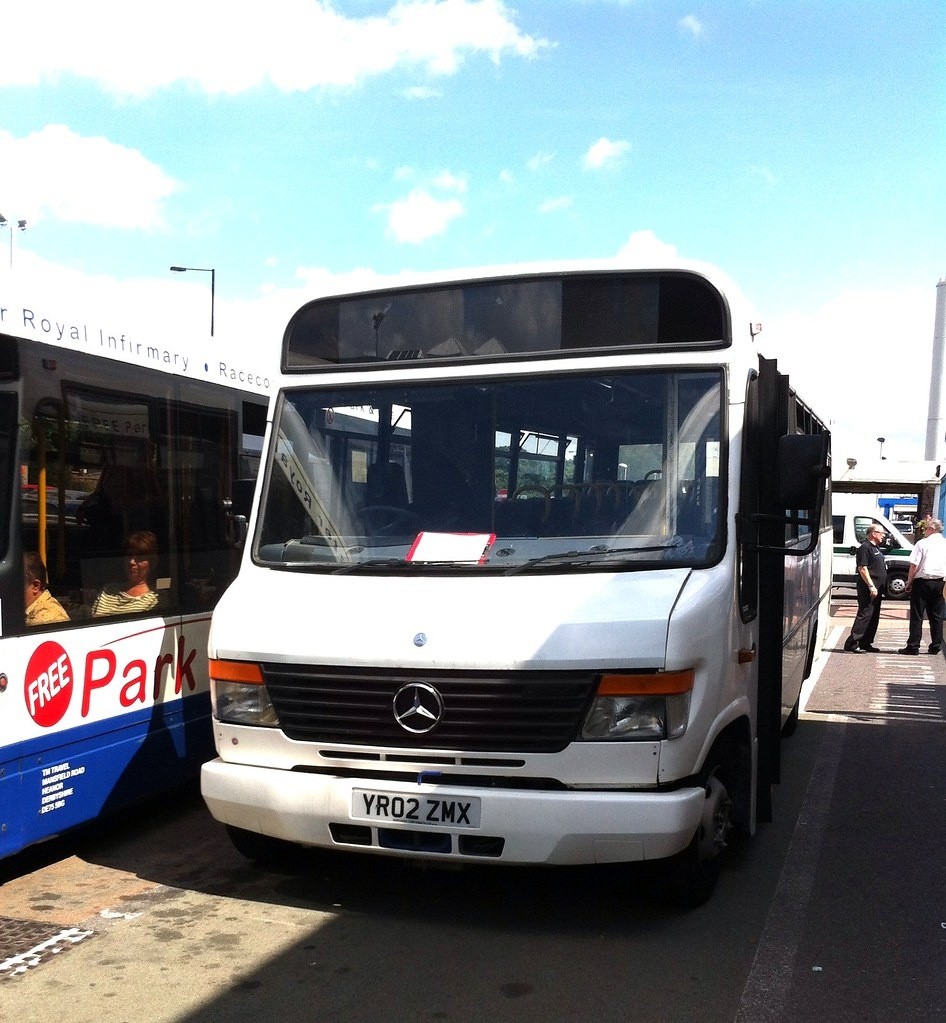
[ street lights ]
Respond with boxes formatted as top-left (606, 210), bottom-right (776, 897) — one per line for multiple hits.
top-left (0, 214), bottom-right (27, 275)
top-left (170, 266), bottom-right (215, 337)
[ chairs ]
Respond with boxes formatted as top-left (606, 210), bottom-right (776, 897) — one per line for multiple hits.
top-left (493, 470), bottom-right (719, 539)
top-left (61, 603), bottom-right (92, 622)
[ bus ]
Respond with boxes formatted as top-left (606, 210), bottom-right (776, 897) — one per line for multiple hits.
top-left (0, 297), bottom-right (412, 861)
top-left (200, 267), bottom-right (831, 908)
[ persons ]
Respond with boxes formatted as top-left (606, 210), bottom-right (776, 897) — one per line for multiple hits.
top-left (898, 517), bottom-right (946, 656)
top-left (19, 552), bottom-right (72, 627)
top-left (87, 529), bottom-right (169, 618)
top-left (843, 523), bottom-right (889, 654)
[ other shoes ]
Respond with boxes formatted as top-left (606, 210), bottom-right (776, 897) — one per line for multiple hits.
top-left (844, 646), bottom-right (866, 653)
top-left (860, 646), bottom-right (879, 652)
top-left (898, 646), bottom-right (919, 655)
top-left (928, 646), bottom-right (941, 654)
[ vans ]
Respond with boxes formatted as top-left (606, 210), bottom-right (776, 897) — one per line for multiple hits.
top-left (832, 507), bottom-right (914, 601)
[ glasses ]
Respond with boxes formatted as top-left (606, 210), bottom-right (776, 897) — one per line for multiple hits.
top-left (874, 531), bottom-right (883, 535)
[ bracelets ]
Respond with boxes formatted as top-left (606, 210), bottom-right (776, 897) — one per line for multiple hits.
top-left (870, 585), bottom-right (874, 587)
